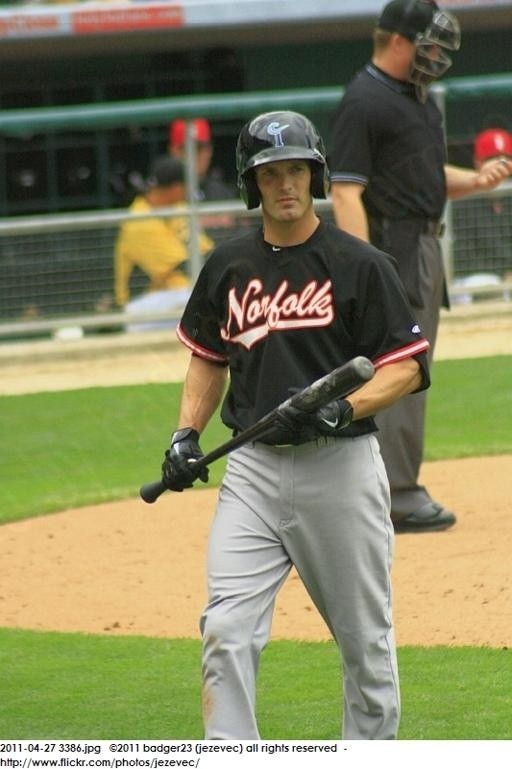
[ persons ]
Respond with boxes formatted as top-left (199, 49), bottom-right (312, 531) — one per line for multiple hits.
top-left (328, 0), bottom-right (512, 530)
top-left (451, 128), bottom-right (512, 308)
top-left (106, 116), bottom-right (215, 332)
top-left (162, 112), bottom-right (430, 739)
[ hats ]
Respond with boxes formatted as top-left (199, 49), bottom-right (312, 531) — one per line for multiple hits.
top-left (147, 156), bottom-right (187, 185)
top-left (169, 119), bottom-right (209, 144)
top-left (473, 128), bottom-right (512, 161)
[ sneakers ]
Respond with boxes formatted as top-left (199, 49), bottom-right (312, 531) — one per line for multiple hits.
top-left (393, 500), bottom-right (457, 531)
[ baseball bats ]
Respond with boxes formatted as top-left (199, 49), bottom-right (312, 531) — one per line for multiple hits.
top-left (139, 356), bottom-right (375, 502)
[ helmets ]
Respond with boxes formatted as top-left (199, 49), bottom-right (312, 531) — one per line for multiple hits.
top-left (378, 0), bottom-right (439, 41)
top-left (234, 110), bottom-right (332, 210)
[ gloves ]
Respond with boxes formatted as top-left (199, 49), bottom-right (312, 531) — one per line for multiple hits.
top-left (275, 386), bottom-right (354, 446)
top-left (161, 427), bottom-right (209, 492)
